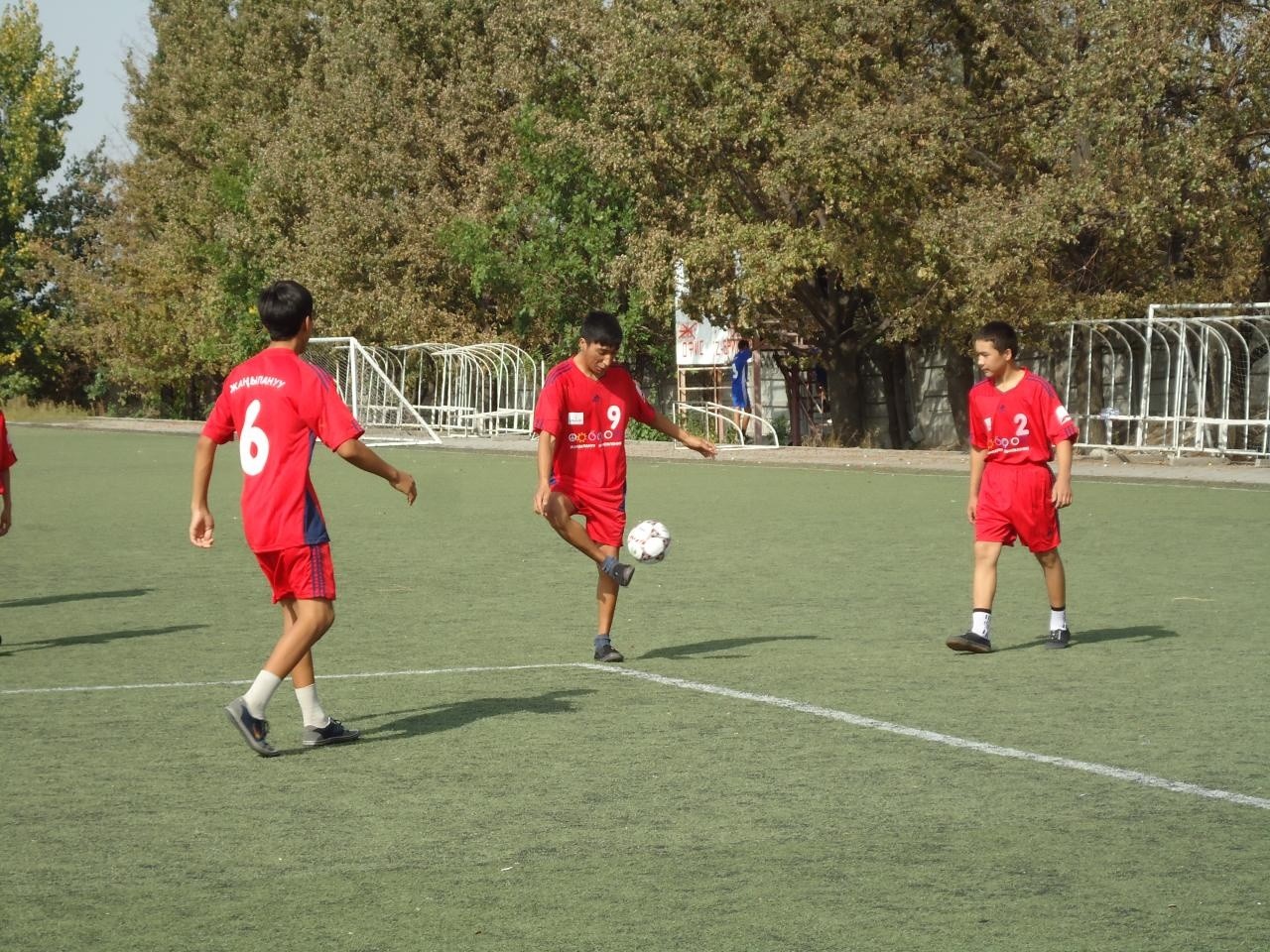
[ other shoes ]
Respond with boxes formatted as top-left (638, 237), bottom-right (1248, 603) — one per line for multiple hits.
top-left (744, 436), bottom-right (754, 445)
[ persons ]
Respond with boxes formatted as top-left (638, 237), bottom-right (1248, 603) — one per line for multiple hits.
top-left (188, 280), bottom-right (418, 756)
top-left (732, 330), bottom-right (760, 444)
top-left (0, 404), bottom-right (19, 648)
top-left (530, 311), bottom-right (718, 661)
top-left (949, 319), bottom-right (1079, 653)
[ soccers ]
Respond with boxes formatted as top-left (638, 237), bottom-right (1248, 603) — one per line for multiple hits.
top-left (627, 520), bottom-right (672, 564)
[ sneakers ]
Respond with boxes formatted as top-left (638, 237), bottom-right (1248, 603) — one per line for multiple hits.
top-left (946, 631), bottom-right (991, 653)
top-left (609, 562), bottom-right (635, 586)
top-left (224, 696), bottom-right (281, 757)
top-left (594, 644), bottom-right (623, 663)
top-left (1045, 626), bottom-right (1070, 649)
top-left (301, 716), bottom-right (360, 744)
